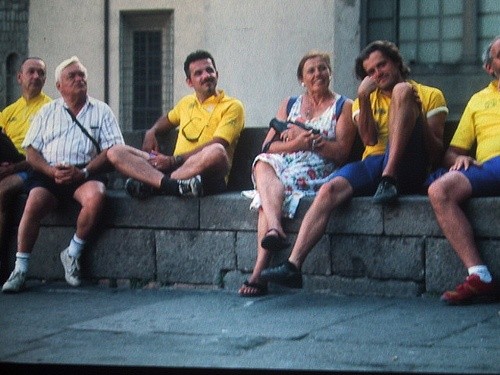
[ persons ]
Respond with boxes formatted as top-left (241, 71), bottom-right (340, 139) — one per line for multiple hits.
top-left (1, 55), bottom-right (126, 293)
top-left (107, 50), bottom-right (244, 199)
top-left (426, 34), bottom-right (500, 306)
top-left (0, 58), bottom-right (54, 244)
top-left (259, 39), bottom-right (448, 290)
top-left (236, 50), bottom-right (355, 297)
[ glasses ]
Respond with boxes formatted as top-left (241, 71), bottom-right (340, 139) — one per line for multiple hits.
top-left (181, 116), bottom-right (208, 144)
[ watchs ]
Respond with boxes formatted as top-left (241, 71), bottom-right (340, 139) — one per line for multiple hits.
top-left (175, 155), bottom-right (183, 168)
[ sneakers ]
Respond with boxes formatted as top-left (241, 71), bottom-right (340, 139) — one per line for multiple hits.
top-left (259, 265), bottom-right (304, 289)
top-left (179, 174), bottom-right (203, 196)
top-left (59, 247), bottom-right (83, 287)
top-left (125, 177), bottom-right (160, 199)
top-left (371, 180), bottom-right (399, 206)
top-left (1, 269), bottom-right (26, 292)
top-left (441, 274), bottom-right (498, 305)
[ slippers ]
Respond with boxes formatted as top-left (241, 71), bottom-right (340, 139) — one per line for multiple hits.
top-left (238, 279), bottom-right (267, 298)
top-left (261, 228), bottom-right (291, 252)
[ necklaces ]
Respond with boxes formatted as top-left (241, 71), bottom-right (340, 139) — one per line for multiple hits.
top-left (304, 110), bottom-right (313, 120)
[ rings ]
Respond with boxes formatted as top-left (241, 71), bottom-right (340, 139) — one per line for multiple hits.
top-left (287, 138), bottom-right (289, 141)
top-left (155, 162), bottom-right (158, 167)
top-left (284, 133), bottom-right (288, 138)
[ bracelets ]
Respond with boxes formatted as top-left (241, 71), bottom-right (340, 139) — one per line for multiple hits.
top-left (82, 167), bottom-right (89, 181)
top-left (169, 156), bottom-right (172, 170)
top-left (415, 125), bottom-right (425, 131)
